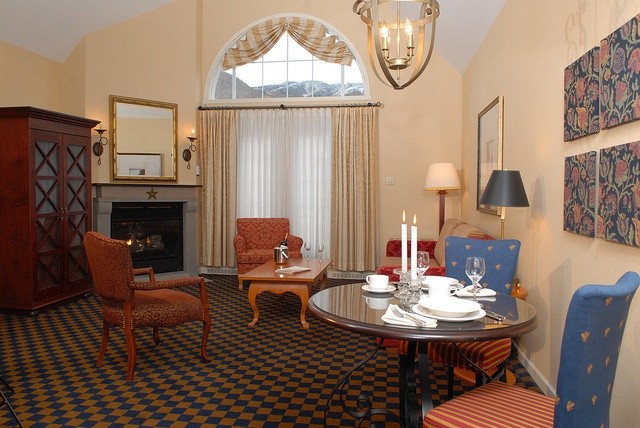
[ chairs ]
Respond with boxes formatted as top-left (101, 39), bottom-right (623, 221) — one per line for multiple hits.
top-left (421, 271), bottom-right (640, 428)
top-left (444, 236), bottom-right (521, 399)
top-left (82, 231), bottom-right (213, 383)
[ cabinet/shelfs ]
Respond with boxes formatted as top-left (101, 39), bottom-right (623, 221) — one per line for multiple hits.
top-left (2, 105), bottom-right (100, 317)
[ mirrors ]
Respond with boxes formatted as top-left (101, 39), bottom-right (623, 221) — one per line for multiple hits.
top-left (109, 95), bottom-right (180, 183)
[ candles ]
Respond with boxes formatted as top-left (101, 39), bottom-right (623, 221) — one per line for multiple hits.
top-left (409, 214), bottom-right (419, 287)
top-left (379, 19), bottom-right (390, 49)
top-left (398, 206), bottom-right (408, 276)
top-left (99, 119), bottom-right (103, 129)
top-left (403, 17), bottom-right (414, 47)
top-left (191, 128), bottom-right (196, 137)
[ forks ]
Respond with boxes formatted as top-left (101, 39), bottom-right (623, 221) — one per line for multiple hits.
top-left (391, 305), bottom-right (424, 326)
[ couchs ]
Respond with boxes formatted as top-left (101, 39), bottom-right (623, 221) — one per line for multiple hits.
top-left (233, 217), bottom-right (304, 291)
top-left (376, 218), bottom-right (490, 346)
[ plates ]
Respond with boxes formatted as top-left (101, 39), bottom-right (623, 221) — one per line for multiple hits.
top-left (454, 285), bottom-right (496, 297)
top-left (418, 295), bottom-right (481, 316)
top-left (421, 283), bottom-right (464, 291)
top-left (416, 275), bottom-right (459, 287)
top-left (362, 284), bottom-right (396, 293)
top-left (412, 303), bottom-right (486, 322)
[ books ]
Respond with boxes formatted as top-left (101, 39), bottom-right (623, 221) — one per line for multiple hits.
top-left (274, 266), bottom-right (311, 275)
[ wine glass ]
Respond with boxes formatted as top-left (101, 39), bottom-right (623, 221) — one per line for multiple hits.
top-left (465, 256), bottom-right (486, 307)
top-left (417, 250), bottom-right (430, 294)
top-left (304, 242), bottom-right (312, 261)
top-left (317, 244), bottom-right (324, 263)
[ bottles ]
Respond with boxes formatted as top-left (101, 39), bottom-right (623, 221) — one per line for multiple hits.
top-left (279, 233), bottom-right (289, 247)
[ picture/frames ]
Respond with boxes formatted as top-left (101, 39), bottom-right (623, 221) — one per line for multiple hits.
top-left (118, 150), bottom-right (165, 177)
top-left (476, 94), bottom-right (506, 217)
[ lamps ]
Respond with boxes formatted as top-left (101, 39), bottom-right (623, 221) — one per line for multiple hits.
top-left (423, 160), bottom-right (461, 239)
top-left (481, 170), bottom-right (531, 241)
top-left (352, 1), bottom-right (442, 91)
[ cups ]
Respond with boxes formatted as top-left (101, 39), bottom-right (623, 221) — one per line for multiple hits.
top-left (429, 282), bottom-right (457, 296)
top-left (366, 274), bottom-right (389, 289)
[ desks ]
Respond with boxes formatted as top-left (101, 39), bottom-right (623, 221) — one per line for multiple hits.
top-left (306, 277), bottom-right (538, 427)
top-left (454, 284), bottom-right (528, 386)
